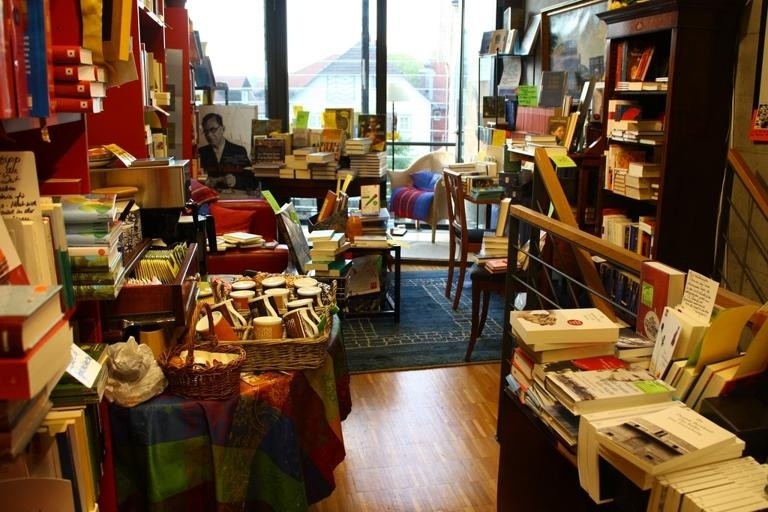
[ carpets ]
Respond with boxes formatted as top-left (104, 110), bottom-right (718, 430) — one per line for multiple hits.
top-left (339, 267), bottom-right (514, 373)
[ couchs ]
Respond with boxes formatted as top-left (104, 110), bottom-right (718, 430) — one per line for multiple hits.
top-left (203, 197), bottom-right (291, 277)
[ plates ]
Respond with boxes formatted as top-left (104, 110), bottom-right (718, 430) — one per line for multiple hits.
top-left (87, 146), bottom-right (117, 169)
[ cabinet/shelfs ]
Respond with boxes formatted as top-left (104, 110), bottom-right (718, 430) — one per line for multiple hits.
top-left (338, 230), bottom-right (401, 324)
top-left (1, 0), bottom-right (199, 510)
top-left (464, 1), bottom-right (743, 333)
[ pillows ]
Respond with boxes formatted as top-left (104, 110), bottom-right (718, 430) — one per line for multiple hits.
top-left (409, 168), bottom-right (442, 191)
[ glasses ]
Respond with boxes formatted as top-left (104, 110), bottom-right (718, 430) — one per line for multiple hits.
top-left (201, 125), bottom-right (221, 136)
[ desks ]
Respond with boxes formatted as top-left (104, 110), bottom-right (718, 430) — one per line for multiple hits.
top-left (97, 275), bottom-right (340, 507)
top-left (259, 177), bottom-right (387, 209)
top-left (498, 388), bottom-right (768, 511)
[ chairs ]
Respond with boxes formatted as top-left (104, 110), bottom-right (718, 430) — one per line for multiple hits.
top-left (444, 169), bottom-right (508, 308)
top-left (464, 162), bottom-right (575, 363)
top-left (387, 150), bottom-right (458, 244)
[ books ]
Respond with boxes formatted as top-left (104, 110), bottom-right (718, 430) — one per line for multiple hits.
top-left (511, 255), bottom-right (768, 512)
top-left (2, 1), bottom-right (199, 510)
top-left (200, 105), bottom-right (390, 285)
top-left (442, 43), bottom-right (673, 274)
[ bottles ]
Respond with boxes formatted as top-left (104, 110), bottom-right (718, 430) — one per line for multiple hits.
top-left (485, 157), bottom-right (498, 177)
top-left (346, 209), bottom-right (364, 241)
top-left (139, 324), bottom-right (166, 360)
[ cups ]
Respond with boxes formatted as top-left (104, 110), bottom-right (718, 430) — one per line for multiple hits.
top-left (280, 307), bottom-right (320, 339)
top-left (252, 316), bottom-right (285, 340)
top-left (230, 276), bottom-right (325, 315)
top-left (195, 311), bottom-right (239, 341)
top-left (247, 295), bottom-right (281, 320)
top-left (285, 297), bottom-right (322, 325)
top-left (115, 221), bottom-right (137, 254)
top-left (201, 298), bottom-right (248, 329)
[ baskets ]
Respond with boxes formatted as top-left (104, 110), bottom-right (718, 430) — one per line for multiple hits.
top-left (156, 300), bottom-right (248, 403)
top-left (183, 276), bottom-right (339, 374)
